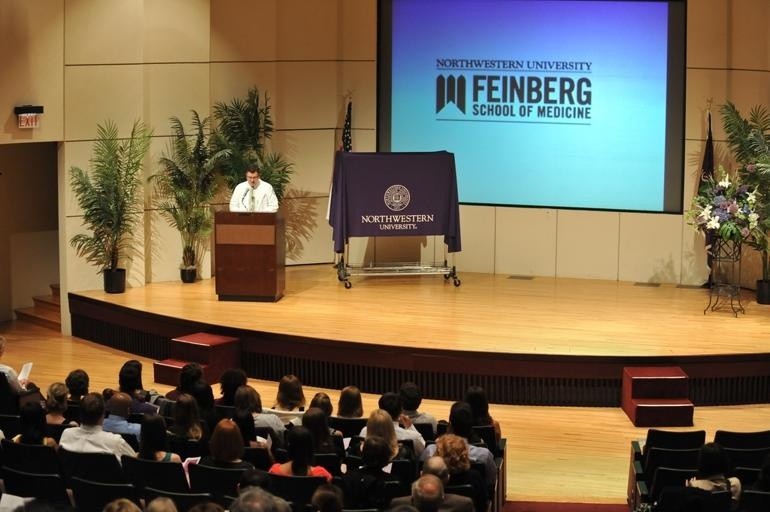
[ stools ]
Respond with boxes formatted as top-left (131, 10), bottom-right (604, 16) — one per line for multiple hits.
top-left (704, 224), bottom-right (745, 318)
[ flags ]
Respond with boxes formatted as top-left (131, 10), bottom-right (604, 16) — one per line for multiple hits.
top-left (698, 114), bottom-right (714, 268)
top-left (339, 101), bottom-right (352, 152)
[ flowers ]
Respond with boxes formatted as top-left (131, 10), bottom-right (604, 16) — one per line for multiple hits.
top-left (684, 164), bottom-right (766, 245)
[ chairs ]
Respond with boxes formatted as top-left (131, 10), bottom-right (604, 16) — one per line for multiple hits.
top-left (625, 429), bottom-right (770, 512)
top-left (0, 395), bottom-right (507, 512)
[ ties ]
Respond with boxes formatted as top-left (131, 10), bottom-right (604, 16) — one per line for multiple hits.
top-left (249, 188), bottom-right (255, 212)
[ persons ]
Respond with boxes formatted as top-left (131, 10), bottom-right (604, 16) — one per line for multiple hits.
top-left (9, 368), bottom-right (141, 469)
top-left (684, 443), bottom-right (742, 512)
top-left (229, 164), bottom-right (279, 214)
top-left (216, 368), bottom-right (364, 512)
top-left (751, 453), bottom-right (770, 492)
top-left (104, 360), bottom-right (252, 512)
top-left (347, 383), bottom-right (500, 512)
top-left (0, 336), bottom-right (46, 401)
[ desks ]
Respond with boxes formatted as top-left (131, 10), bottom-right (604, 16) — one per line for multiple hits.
top-left (326, 151), bottom-right (461, 289)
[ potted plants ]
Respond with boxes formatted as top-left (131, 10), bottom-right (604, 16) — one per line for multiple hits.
top-left (718, 99), bottom-right (770, 304)
top-left (67, 118), bottom-right (158, 295)
top-left (148, 109), bottom-right (232, 283)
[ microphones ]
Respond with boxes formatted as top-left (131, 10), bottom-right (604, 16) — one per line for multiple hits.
top-left (242, 188), bottom-right (250, 212)
